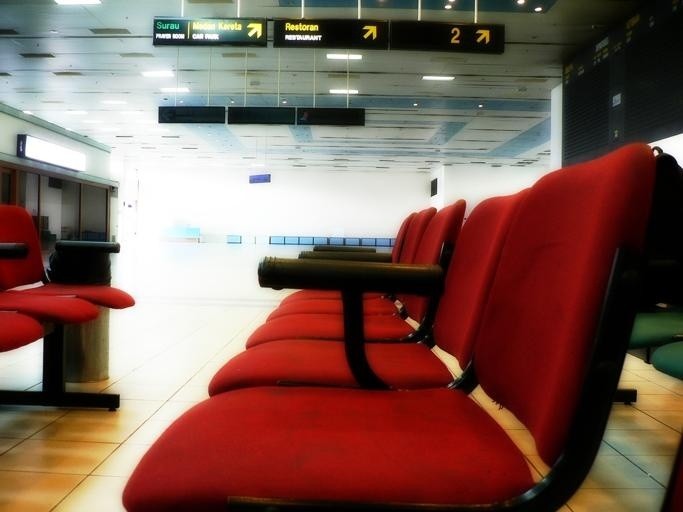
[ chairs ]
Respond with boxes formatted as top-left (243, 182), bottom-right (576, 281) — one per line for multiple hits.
top-left (0, 202), bottom-right (136, 415)
top-left (120, 138), bottom-right (655, 511)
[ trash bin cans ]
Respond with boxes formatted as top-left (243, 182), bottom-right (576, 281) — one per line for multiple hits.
top-left (49, 239), bottom-right (120, 384)
